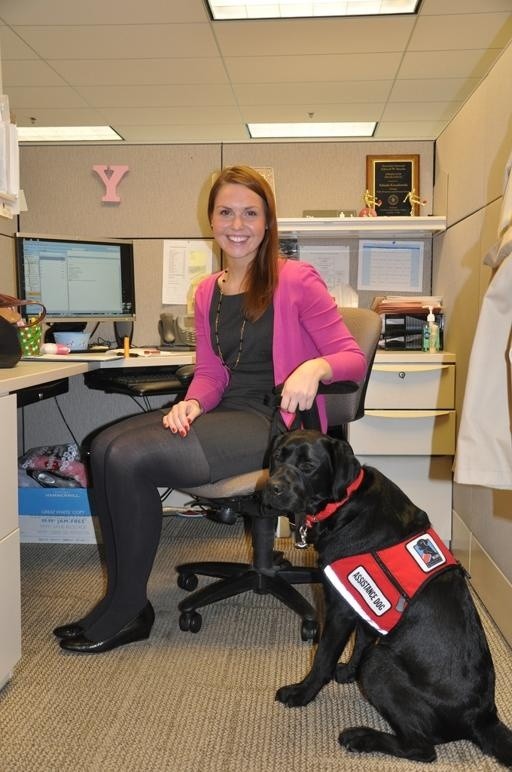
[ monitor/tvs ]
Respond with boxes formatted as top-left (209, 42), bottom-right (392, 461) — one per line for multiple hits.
top-left (15, 232), bottom-right (137, 353)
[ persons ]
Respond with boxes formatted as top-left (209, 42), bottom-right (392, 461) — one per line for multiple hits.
top-left (53, 163), bottom-right (368, 652)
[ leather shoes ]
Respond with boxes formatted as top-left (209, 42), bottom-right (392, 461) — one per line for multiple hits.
top-left (60, 598), bottom-right (155, 652)
top-left (54, 622), bottom-right (87, 637)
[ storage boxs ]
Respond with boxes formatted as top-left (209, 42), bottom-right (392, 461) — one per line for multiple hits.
top-left (18, 488), bottom-right (101, 516)
top-left (18, 516), bottom-right (103, 545)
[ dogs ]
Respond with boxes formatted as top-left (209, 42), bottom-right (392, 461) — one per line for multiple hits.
top-left (263, 428), bottom-right (512, 772)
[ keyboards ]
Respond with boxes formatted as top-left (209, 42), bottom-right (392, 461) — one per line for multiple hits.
top-left (108, 373), bottom-right (178, 384)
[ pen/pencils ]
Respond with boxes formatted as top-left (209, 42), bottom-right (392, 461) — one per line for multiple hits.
top-left (26, 312), bottom-right (43, 327)
top-left (117, 352), bottom-right (140, 358)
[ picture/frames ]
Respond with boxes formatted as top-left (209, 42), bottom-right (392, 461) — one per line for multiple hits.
top-left (366, 154), bottom-right (421, 217)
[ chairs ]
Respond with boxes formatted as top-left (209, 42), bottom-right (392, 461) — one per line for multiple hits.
top-left (175, 308), bottom-right (382, 645)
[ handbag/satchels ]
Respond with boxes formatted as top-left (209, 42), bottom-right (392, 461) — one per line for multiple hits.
top-left (1, 293), bottom-right (47, 368)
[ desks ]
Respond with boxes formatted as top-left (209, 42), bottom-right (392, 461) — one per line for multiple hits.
top-left (1, 347), bottom-right (196, 693)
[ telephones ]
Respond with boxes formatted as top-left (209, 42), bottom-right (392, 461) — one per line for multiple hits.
top-left (158, 312), bottom-right (196, 352)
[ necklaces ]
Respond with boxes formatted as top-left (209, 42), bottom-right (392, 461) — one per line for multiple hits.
top-left (214, 267), bottom-right (246, 370)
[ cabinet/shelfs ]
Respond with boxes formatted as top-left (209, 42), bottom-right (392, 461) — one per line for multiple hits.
top-left (345, 352), bottom-right (459, 554)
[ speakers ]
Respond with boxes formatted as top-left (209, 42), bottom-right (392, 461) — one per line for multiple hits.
top-left (113, 321), bottom-right (138, 349)
